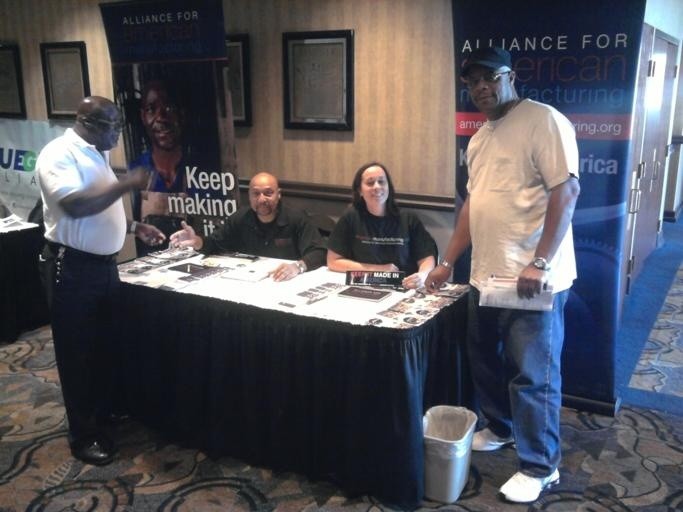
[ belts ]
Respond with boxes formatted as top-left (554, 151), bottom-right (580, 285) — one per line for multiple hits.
top-left (51, 242), bottom-right (118, 261)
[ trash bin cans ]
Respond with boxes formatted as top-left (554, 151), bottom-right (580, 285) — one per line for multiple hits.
top-left (422, 405), bottom-right (478, 504)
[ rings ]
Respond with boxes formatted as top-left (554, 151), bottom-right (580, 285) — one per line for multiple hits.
top-left (413, 280), bottom-right (417, 284)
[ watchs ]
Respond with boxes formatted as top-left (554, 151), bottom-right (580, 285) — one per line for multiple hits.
top-left (531, 257), bottom-right (551, 271)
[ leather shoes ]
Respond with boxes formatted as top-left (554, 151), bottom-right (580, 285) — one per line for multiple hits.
top-left (73, 440), bottom-right (112, 465)
top-left (99, 406), bottom-right (133, 423)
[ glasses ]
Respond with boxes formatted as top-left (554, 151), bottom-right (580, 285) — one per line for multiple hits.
top-left (99, 119), bottom-right (126, 131)
top-left (466, 71), bottom-right (511, 89)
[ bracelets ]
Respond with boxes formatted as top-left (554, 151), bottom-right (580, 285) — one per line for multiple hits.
top-left (294, 261), bottom-right (304, 273)
top-left (129, 220), bottom-right (139, 235)
top-left (436, 257), bottom-right (453, 271)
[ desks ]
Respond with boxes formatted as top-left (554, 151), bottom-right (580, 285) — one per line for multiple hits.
top-left (0, 216), bottom-right (48, 345)
top-left (115, 242), bottom-right (471, 492)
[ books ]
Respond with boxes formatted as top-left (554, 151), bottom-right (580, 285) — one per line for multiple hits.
top-left (336, 286), bottom-right (391, 302)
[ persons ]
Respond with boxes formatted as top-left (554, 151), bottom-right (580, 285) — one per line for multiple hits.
top-left (169, 171), bottom-right (327, 282)
top-left (421, 44), bottom-right (584, 505)
top-left (128, 75), bottom-right (221, 252)
top-left (324, 161), bottom-right (438, 290)
top-left (32, 92), bottom-right (166, 465)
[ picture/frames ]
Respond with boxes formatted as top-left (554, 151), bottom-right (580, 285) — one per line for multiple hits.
top-left (225, 27), bottom-right (357, 134)
top-left (1, 42), bottom-right (91, 121)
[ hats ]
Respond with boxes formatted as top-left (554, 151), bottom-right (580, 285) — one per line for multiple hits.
top-left (462, 45), bottom-right (512, 81)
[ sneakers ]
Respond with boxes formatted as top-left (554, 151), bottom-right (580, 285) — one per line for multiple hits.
top-left (499, 466), bottom-right (560, 503)
top-left (471, 428), bottom-right (517, 451)
